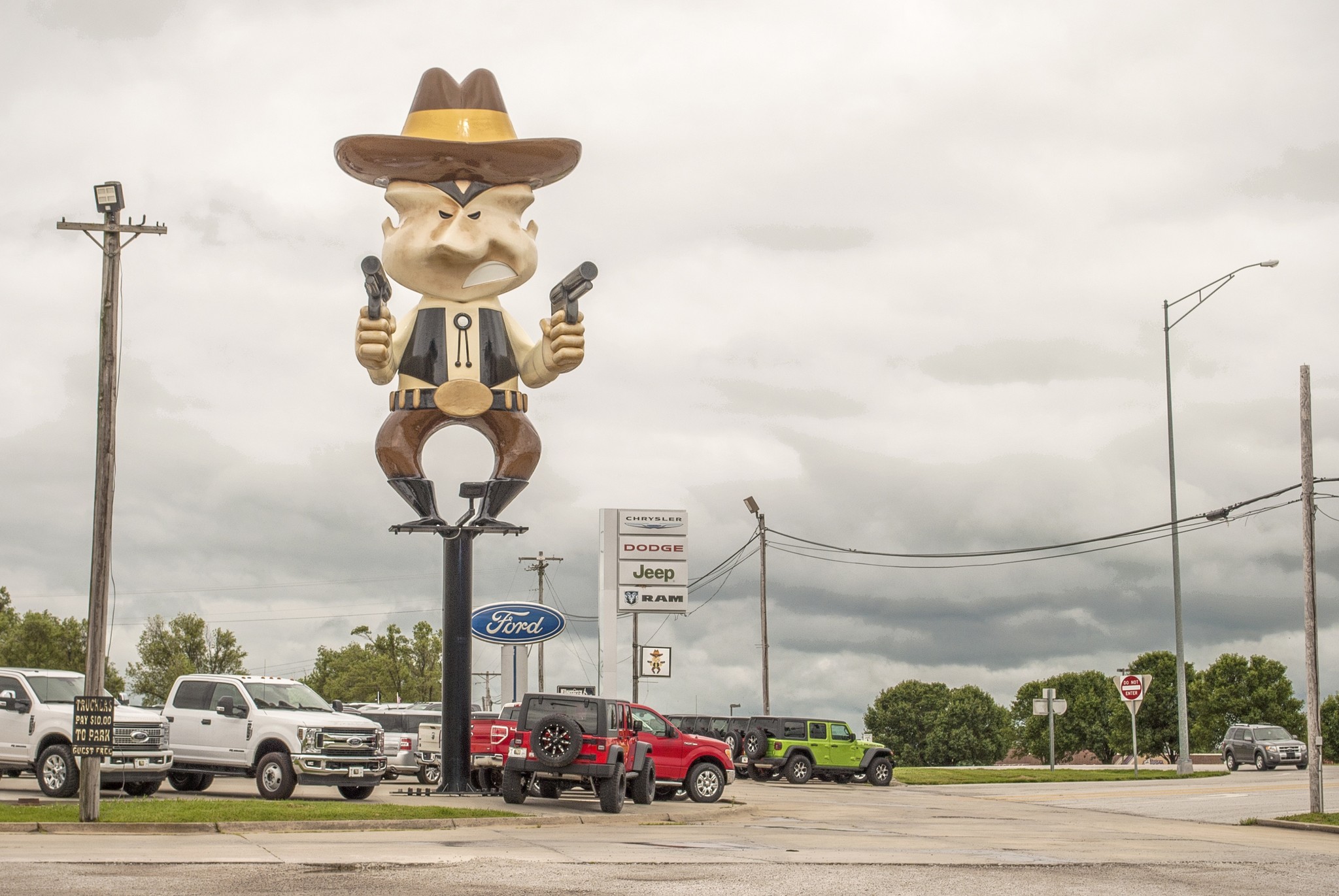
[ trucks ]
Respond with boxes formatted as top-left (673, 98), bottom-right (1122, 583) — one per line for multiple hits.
top-left (128, 673), bottom-right (388, 801)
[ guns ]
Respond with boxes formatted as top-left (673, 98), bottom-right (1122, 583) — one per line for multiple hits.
top-left (361, 255), bottom-right (392, 320)
top-left (549, 261), bottom-right (599, 325)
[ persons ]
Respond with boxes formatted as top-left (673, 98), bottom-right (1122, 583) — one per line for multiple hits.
top-left (334, 66), bottom-right (585, 528)
top-left (648, 649), bottom-right (663, 674)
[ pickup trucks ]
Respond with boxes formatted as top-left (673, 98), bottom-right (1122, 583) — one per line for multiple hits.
top-left (468, 702), bottom-right (736, 804)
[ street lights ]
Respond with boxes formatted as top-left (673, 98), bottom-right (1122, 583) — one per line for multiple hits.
top-left (730, 704), bottom-right (740, 716)
top-left (742, 496), bottom-right (770, 716)
top-left (54, 179), bottom-right (167, 822)
top-left (1164, 260), bottom-right (1282, 773)
top-left (1116, 668), bottom-right (1125, 676)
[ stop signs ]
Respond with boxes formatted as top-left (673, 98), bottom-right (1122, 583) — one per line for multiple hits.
top-left (1120, 675), bottom-right (1143, 701)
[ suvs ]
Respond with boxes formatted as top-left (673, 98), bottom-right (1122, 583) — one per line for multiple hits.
top-left (660, 713), bottom-right (852, 784)
top-left (501, 692), bottom-right (656, 814)
top-left (744, 715), bottom-right (897, 787)
top-left (328, 700), bottom-right (500, 791)
top-left (0, 667), bottom-right (174, 798)
top-left (1221, 722), bottom-right (1309, 772)
